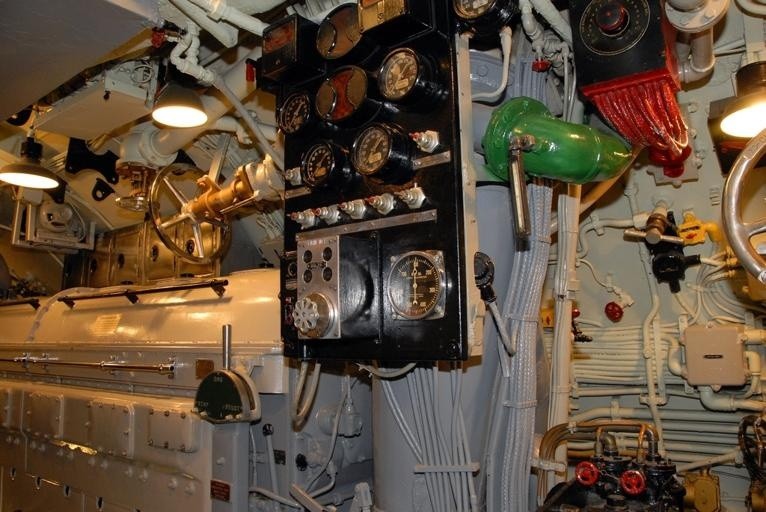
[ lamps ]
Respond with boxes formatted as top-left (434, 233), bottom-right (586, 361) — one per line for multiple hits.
top-left (0, 126), bottom-right (60, 190)
top-left (150, 84), bottom-right (209, 130)
top-left (720, 60), bottom-right (766, 139)
